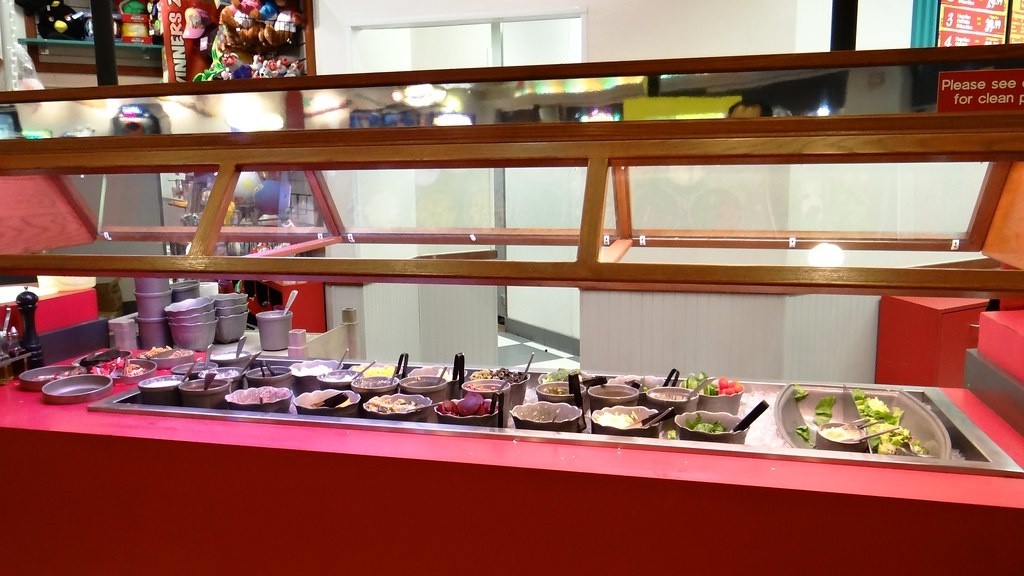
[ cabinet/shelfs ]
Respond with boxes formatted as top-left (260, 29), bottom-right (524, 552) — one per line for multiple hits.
top-left (874, 293), bottom-right (992, 391)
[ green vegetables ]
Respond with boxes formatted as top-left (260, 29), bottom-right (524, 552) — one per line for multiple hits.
top-left (687, 414), bottom-right (726, 432)
top-left (814, 396), bottom-right (835, 426)
top-left (794, 385), bottom-right (807, 401)
top-left (852, 388), bottom-right (928, 455)
top-left (796, 426), bottom-right (813, 449)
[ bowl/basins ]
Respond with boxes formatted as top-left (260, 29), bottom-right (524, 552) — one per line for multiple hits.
top-left (591, 405), bottom-right (661, 439)
top-left (434, 399), bottom-right (500, 426)
top-left (225, 386), bottom-right (292, 413)
top-left (363, 394), bottom-right (433, 421)
top-left (407, 364), bottom-right (468, 392)
top-left (292, 389), bottom-right (361, 418)
top-left (71, 350), bottom-right (133, 372)
top-left (511, 402), bottom-right (583, 432)
top-left (348, 362), bottom-right (405, 379)
top-left (675, 411), bottom-right (748, 444)
top-left (136, 349), bottom-right (195, 370)
top-left (164, 293), bottom-right (249, 351)
top-left (289, 359), bottom-right (343, 396)
top-left (679, 378), bottom-right (745, 415)
top-left (41, 374), bottom-right (113, 404)
top-left (106, 358), bottom-right (158, 385)
top-left (18, 365), bottom-right (87, 392)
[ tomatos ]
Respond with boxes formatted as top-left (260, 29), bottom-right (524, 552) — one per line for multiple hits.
top-left (719, 377), bottom-right (742, 394)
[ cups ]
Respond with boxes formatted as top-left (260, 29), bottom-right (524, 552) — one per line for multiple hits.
top-left (351, 375), bottom-right (399, 402)
top-left (646, 386), bottom-right (700, 429)
top-left (399, 376), bottom-right (447, 402)
top-left (587, 385), bottom-right (639, 409)
top-left (536, 382), bottom-right (587, 408)
top-left (256, 310), bottom-right (294, 351)
top-left (170, 281), bottom-right (200, 301)
top-left (132, 291), bottom-right (173, 318)
top-left (246, 366), bottom-right (292, 391)
top-left (317, 370), bottom-right (364, 391)
top-left (138, 375), bottom-right (189, 406)
top-left (134, 277), bottom-right (169, 292)
top-left (133, 316), bottom-right (167, 349)
top-left (177, 379), bottom-right (231, 409)
top-left (209, 350), bottom-right (252, 371)
top-left (462, 379), bottom-right (511, 412)
top-left (815, 423), bottom-right (867, 452)
top-left (170, 362), bottom-right (218, 380)
top-left (200, 366), bottom-right (246, 392)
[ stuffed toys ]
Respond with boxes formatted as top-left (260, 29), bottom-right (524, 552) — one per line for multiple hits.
top-left (37, 1), bottom-right (86, 42)
top-left (213, 0), bottom-right (305, 80)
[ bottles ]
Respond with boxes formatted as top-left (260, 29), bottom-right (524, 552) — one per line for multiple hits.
top-left (15, 291), bottom-right (44, 369)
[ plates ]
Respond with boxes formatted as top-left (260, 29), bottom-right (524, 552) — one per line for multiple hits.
top-left (470, 368), bottom-right (531, 385)
top-left (605, 376), bottom-right (672, 399)
top-left (537, 370), bottom-right (589, 385)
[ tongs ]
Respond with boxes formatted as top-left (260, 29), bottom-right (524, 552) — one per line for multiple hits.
top-left (625, 408), bottom-right (674, 428)
top-left (260, 360), bottom-right (275, 377)
top-left (453, 352), bottom-right (465, 400)
top-left (568, 371), bottom-right (591, 433)
top-left (491, 390), bottom-right (504, 429)
top-left (397, 352), bottom-right (409, 378)
top-left (311, 392), bottom-right (349, 408)
